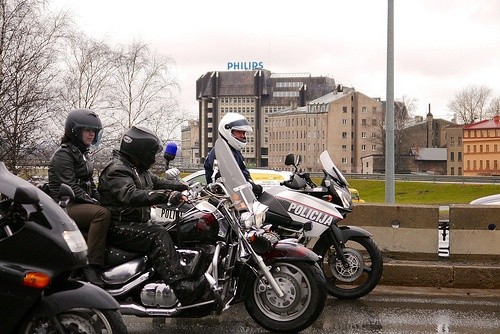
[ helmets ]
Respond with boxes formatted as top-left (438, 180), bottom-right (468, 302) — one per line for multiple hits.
top-left (63, 109), bottom-right (102, 151)
top-left (120, 126), bottom-right (163, 172)
top-left (218, 113), bottom-right (252, 151)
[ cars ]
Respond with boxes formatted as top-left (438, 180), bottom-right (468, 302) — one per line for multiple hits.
top-left (349, 188), bottom-right (365, 204)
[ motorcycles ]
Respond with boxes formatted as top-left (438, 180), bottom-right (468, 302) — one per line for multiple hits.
top-left (0, 158), bottom-right (128, 334)
top-left (162, 141), bottom-right (384, 301)
top-left (36, 137), bottom-right (328, 333)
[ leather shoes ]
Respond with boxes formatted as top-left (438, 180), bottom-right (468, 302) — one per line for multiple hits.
top-left (79, 264), bottom-right (108, 288)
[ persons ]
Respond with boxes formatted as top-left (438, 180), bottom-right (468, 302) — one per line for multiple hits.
top-left (204, 113), bottom-right (263, 207)
top-left (47, 108), bottom-right (112, 288)
top-left (97, 124), bottom-right (210, 303)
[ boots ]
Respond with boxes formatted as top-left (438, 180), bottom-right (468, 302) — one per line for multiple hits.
top-left (170, 276), bottom-right (210, 306)
